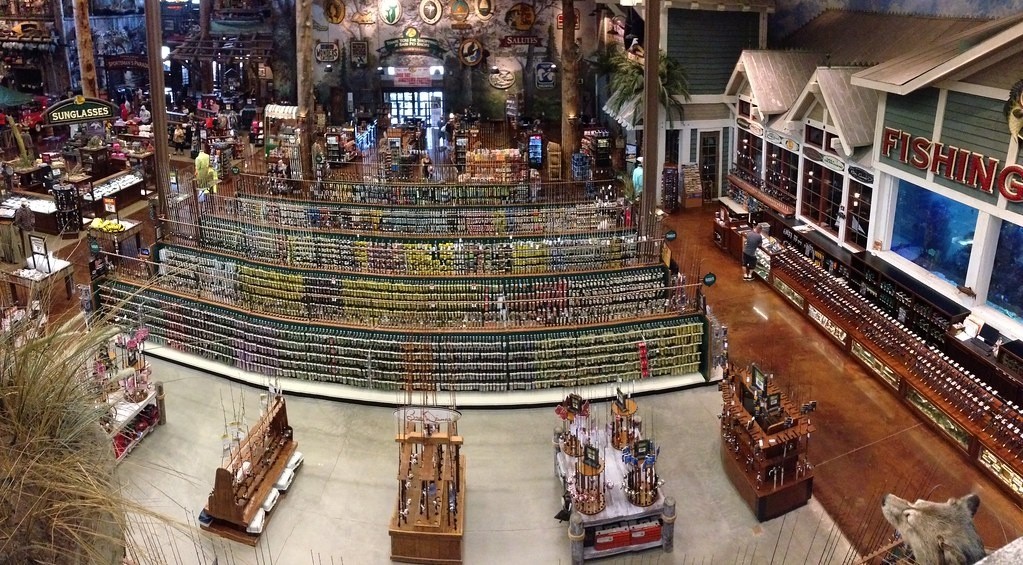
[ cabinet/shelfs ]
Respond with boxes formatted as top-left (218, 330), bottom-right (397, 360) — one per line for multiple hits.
top-left (0, 85), bottom-right (1022, 565)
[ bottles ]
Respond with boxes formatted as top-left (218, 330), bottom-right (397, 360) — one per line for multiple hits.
top-left (768, 243), bottom-right (1023, 466)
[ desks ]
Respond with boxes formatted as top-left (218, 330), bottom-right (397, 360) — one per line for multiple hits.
top-left (90, 215), bottom-right (144, 258)
top-left (1, 253), bottom-right (74, 313)
top-left (148, 190), bottom-right (194, 224)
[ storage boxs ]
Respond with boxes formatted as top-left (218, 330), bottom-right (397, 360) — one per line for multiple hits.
top-left (546, 147), bottom-right (561, 181)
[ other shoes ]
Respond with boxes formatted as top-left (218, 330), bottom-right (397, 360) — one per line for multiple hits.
top-left (173, 152), bottom-right (177, 155)
top-left (743, 277), bottom-right (747, 280)
top-left (180, 152), bottom-right (184, 155)
top-left (747, 276), bottom-right (755, 280)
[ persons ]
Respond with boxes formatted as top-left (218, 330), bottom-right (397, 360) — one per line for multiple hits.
top-left (422, 153), bottom-right (433, 178)
top-left (247, 126), bottom-right (256, 156)
top-left (633, 156), bottom-right (643, 197)
top-left (1, 86), bottom-right (150, 156)
top-left (15, 198), bottom-right (35, 232)
top-left (195, 150), bottom-right (209, 185)
top-left (741, 225), bottom-right (762, 281)
top-left (173, 124), bottom-right (185, 155)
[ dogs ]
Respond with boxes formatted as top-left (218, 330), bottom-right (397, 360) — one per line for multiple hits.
top-left (881, 493), bottom-right (986, 565)
